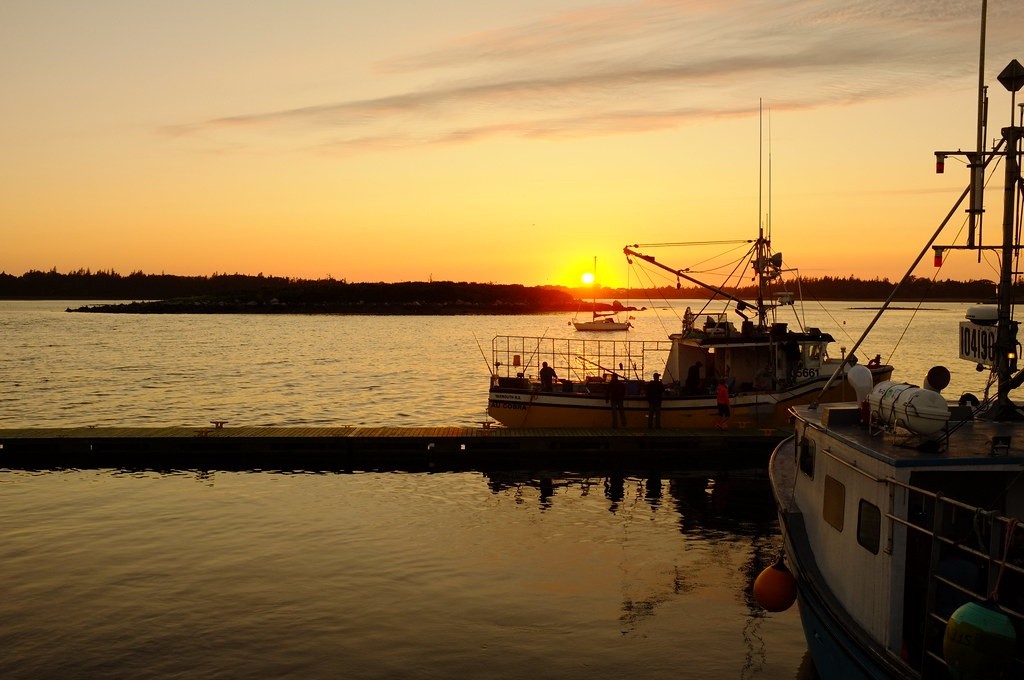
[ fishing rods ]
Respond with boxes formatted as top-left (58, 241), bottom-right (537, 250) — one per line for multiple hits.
top-left (658, 354), bottom-right (675, 382)
top-left (471, 330), bottom-right (493, 375)
top-left (496, 331), bottom-right (498, 375)
top-left (516, 326), bottom-right (550, 378)
top-left (557, 348), bottom-right (590, 394)
top-left (623, 341), bottom-right (640, 381)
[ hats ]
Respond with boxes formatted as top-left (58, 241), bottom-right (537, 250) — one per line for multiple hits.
top-left (653, 373), bottom-right (661, 377)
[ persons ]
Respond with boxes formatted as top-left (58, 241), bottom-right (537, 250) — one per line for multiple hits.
top-left (784, 333), bottom-right (801, 383)
top-left (686, 361), bottom-right (703, 395)
top-left (715, 376), bottom-right (731, 430)
top-left (540, 362), bottom-right (558, 391)
top-left (605, 373), bottom-right (626, 430)
top-left (645, 373), bottom-right (664, 430)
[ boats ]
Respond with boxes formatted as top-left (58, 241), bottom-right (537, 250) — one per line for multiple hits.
top-left (767, 0), bottom-right (1024, 680)
top-left (487, 96), bottom-right (896, 430)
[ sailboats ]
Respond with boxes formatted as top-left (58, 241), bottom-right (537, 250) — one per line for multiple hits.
top-left (574, 256), bottom-right (631, 331)
